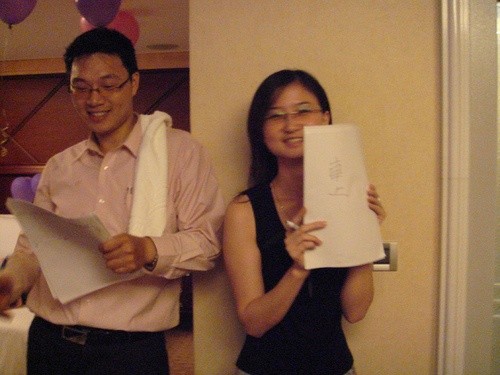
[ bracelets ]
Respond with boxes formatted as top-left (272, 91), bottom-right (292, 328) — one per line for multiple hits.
top-left (146, 255), bottom-right (157, 270)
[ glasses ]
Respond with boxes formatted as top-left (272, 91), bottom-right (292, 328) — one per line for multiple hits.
top-left (267, 108), bottom-right (323, 124)
top-left (67, 75), bottom-right (132, 97)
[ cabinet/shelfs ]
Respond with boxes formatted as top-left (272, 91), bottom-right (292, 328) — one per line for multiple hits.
top-left (0, 50), bottom-right (190, 215)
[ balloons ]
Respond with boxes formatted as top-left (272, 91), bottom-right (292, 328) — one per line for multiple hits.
top-left (0, 0), bottom-right (38, 29)
top-left (75, 0), bottom-right (140, 48)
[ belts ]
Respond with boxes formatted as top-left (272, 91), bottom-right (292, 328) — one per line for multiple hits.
top-left (39, 319), bottom-right (141, 346)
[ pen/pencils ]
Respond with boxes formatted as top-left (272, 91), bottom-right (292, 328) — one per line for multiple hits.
top-left (285, 220), bottom-right (299, 230)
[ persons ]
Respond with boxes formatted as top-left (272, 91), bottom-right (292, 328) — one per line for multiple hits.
top-left (221, 66), bottom-right (389, 375)
top-left (0, 25), bottom-right (226, 375)
